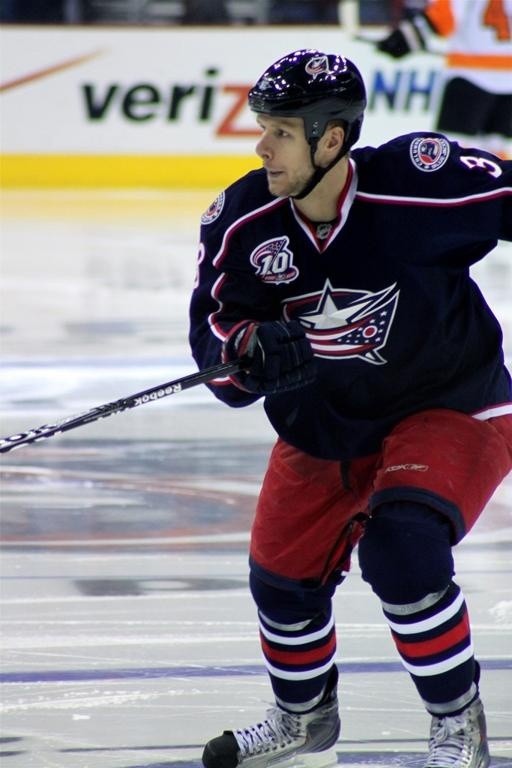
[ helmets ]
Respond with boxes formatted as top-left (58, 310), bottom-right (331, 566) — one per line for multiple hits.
top-left (247, 48), bottom-right (365, 138)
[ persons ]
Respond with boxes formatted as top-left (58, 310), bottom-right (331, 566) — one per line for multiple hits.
top-left (158, 42), bottom-right (509, 765)
top-left (378, 3), bottom-right (511, 166)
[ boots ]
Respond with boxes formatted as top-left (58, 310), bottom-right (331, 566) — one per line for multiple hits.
top-left (203, 662), bottom-right (340, 767)
top-left (424, 659), bottom-right (491, 768)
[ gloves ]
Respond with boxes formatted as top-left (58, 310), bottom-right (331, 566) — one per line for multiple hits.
top-left (223, 320), bottom-right (316, 396)
